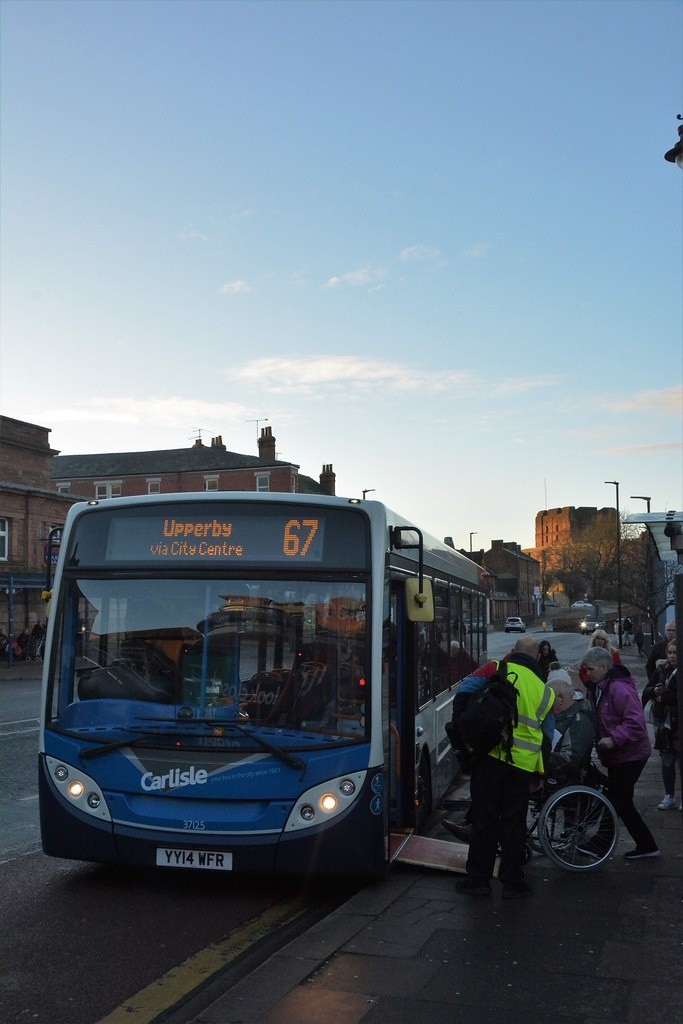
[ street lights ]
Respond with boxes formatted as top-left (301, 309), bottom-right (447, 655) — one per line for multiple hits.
top-left (605, 480), bottom-right (623, 647)
top-left (630, 496), bottom-right (655, 613)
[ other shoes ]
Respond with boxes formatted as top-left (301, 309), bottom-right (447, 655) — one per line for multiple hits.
top-left (560, 827), bottom-right (585, 838)
top-left (657, 794), bottom-right (678, 810)
top-left (627, 643), bottom-right (630, 646)
top-left (575, 841), bottom-right (606, 860)
top-left (622, 643), bottom-right (627, 646)
top-left (455, 877), bottom-right (491, 896)
top-left (624, 845), bottom-right (661, 858)
top-left (501, 885), bottom-right (533, 899)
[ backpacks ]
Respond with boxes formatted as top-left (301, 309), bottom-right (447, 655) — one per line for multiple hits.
top-left (445, 660), bottom-right (520, 775)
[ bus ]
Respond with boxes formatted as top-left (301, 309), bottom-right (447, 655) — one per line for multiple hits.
top-left (37, 478), bottom-right (492, 890)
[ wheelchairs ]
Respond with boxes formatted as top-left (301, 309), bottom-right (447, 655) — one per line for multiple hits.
top-left (456, 736), bottom-right (619, 870)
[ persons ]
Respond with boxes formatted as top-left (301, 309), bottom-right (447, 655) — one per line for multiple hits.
top-left (0, 628), bottom-right (21, 661)
top-left (442, 629), bottom-right (659, 900)
top-left (614, 616), bottom-right (631, 646)
top-left (17, 620), bottom-right (46, 658)
top-left (632, 628), bottom-right (646, 657)
top-left (645, 620), bottom-right (677, 681)
top-left (642, 638), bottom-right (683, 810)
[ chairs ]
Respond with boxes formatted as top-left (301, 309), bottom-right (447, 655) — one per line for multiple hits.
top-left (242, 666), bottom-right (307, 728)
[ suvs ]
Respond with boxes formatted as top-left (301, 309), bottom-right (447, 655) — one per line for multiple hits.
top-left (505, 617), bottom-right (526, 633)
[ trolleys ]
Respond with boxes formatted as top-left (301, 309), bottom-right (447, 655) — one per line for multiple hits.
top-left (25, 633), bottom-right (45, 663)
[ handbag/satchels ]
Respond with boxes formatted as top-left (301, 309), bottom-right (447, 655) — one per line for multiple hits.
top-left (654, 711), bottom-right (673, 753)
top-left (644, 697), bottom-right (659, 725)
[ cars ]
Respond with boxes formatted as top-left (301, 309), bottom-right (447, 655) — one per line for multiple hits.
top-left (581, 614), bottom-right (605, 634)
top-left (572, 601), bottom-right (593, 608)
